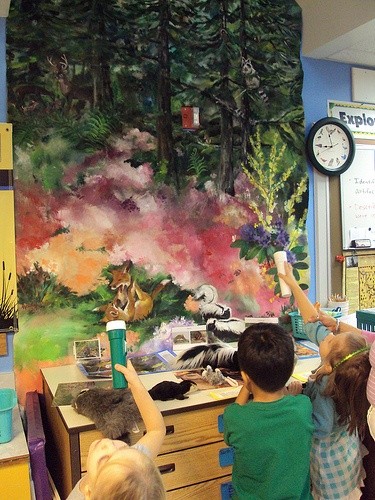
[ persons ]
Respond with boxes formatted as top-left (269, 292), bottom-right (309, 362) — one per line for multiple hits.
top-left (223, 322), bottom-right (314, 500)
top-left (277, 261), bottom-right (375, 500)
top-left (65, 358), bottom-right (167, 500)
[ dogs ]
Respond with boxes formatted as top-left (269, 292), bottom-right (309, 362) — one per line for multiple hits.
top-left (174, 342), bottom-right (239, 370)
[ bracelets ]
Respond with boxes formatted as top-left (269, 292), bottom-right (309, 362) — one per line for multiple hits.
top-left (335, 319), bottom-right (340, 336)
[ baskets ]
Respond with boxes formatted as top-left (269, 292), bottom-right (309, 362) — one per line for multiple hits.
top-left (288, 311), bottom-right (342, 340)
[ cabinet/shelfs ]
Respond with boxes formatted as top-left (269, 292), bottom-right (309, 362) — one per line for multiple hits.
top-left (40, 342), bottom-right (323, 500)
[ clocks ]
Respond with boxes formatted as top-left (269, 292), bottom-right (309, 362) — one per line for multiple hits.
top-left (306, 116), bottom-right (356, 177)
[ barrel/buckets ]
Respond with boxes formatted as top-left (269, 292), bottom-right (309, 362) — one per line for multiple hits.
top-left (328, 299), bottom-right (349, 316)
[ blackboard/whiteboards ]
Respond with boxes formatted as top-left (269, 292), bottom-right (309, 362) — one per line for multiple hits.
top-left (339, 144), bottom-right (375, 251)
